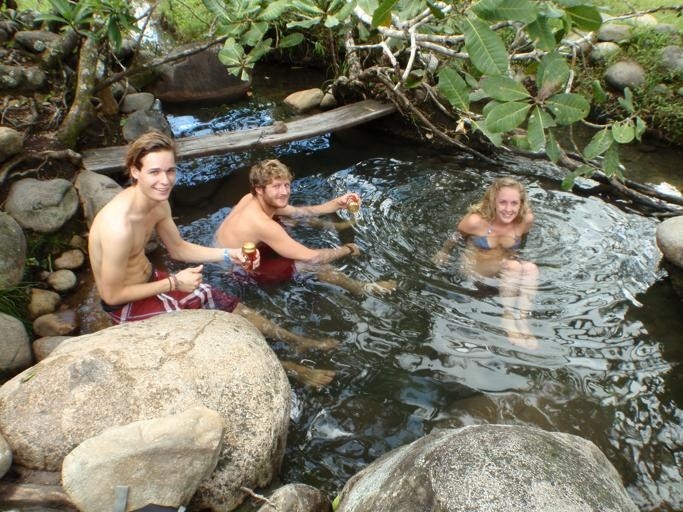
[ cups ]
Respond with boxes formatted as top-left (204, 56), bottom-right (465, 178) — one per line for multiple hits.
top-left (345, 191), bottom-right (361, 214)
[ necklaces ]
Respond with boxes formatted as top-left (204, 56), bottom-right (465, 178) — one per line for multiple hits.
top-left (488, 220), bottom-right (512, 234)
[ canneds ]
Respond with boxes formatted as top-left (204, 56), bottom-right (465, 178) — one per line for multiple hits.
top-left (242, 242), bottom-right (256, 272)
top-left (346, 195), bottom-right (359, 214)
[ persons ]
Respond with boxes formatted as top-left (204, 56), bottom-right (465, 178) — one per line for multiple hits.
top-left (432, 177), bottom-right (540, 351)
top-left (85, 131), bottom-right (340, 389)
top-left (212, 158), bottom-right (399, 297)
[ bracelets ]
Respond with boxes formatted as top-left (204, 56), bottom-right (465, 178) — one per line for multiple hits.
top-left (166, 272), bottom-right (176, 290)
top-left (222, 247), bottom-right (229, 263)
top-left (345, 244), bottom-right (353, 255)
